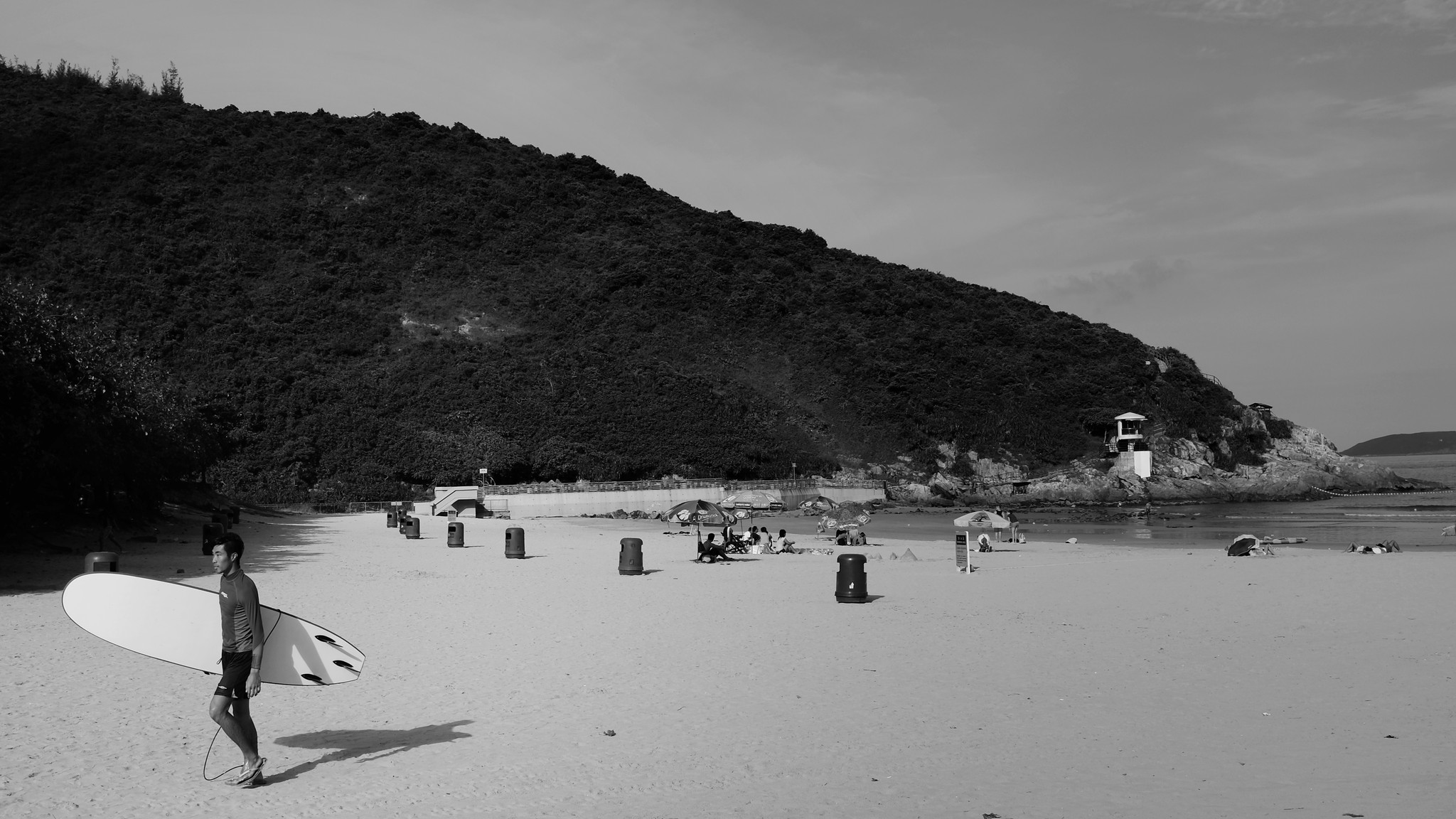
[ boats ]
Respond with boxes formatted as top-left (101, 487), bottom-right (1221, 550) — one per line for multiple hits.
top-left (1259, 537), bottom-right (1308, 544)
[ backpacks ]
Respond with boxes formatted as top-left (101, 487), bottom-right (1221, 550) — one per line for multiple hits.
top-left (1019, 533), bottom-right (1026, 544)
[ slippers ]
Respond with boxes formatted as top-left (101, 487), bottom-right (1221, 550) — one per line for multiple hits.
top-left (225, 777), bottom-right (266, 786)
top-left (235, 757), bottom-right (267, 786)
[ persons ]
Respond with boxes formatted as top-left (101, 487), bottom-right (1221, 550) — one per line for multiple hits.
top-left (698, 533), bottom-right (735, 561)
top-left (993, 505), bottom-right (1005, 543)
top-left (1145, 501), bottom-right (1152, 518)
top-left (1006, 509), bottom-right (1019, 543)
top-left (1341, 540), bottom-right (1403, 554)
top-left (776, 529), bottom-right (800, 554)
top-left (1248, 543), bottom-right (1276, 557)
top-left (207, 533), bottom-right (267, 787)
top-left (742, 526), bottom-right (772, 545)
top-left (835, 528), bottom-right (867, 545)
top-left (977, 528), bottom-right (990, 548)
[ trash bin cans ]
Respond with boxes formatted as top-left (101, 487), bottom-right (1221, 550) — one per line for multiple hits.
top-left (84, 551), bottom-right (120, 574)
top-left (386, 512), bottom-right (397, 528)
top-left (405, 518), bottom-right (420, 539)
top-left (202, 506), bottom-right (241, 555)
top-left (504, 528), bottom-right (525, 559)
top-left (835, 554), bottom-right (867, 603)
top-left (399, 516), bottom-right (411, 535)
top-left (398, 509), bottom-right (407, 522)
top-left (447, 522), bottom-right (464, 547)
top-left (618, 538), bottom-right (644, 575)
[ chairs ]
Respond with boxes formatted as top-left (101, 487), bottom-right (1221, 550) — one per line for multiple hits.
top-left (720, 531), bottom-right (749, 554)
top-left (698, 541), bottom-right (720, 563)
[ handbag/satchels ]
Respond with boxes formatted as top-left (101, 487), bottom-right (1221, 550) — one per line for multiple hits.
top-left (698, 531), bottom-right (705, 552)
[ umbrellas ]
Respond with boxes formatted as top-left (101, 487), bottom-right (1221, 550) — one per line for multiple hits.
top-left (953, 510), bottom-right (1010, 530)
top-left (659, 499), bottom-right (737, 564)
top-left (716, 490), bottom-right (788, 554)
top-left (797, 496), bottom-right (872, 540)
top-left (1227, 534), bottom-right (1260, 556)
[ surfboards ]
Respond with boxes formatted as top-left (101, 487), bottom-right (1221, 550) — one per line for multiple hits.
top-left (62, 571), bottom-right (366, 687)
top-left (1441, 526), bottom-right (1456, 536)
